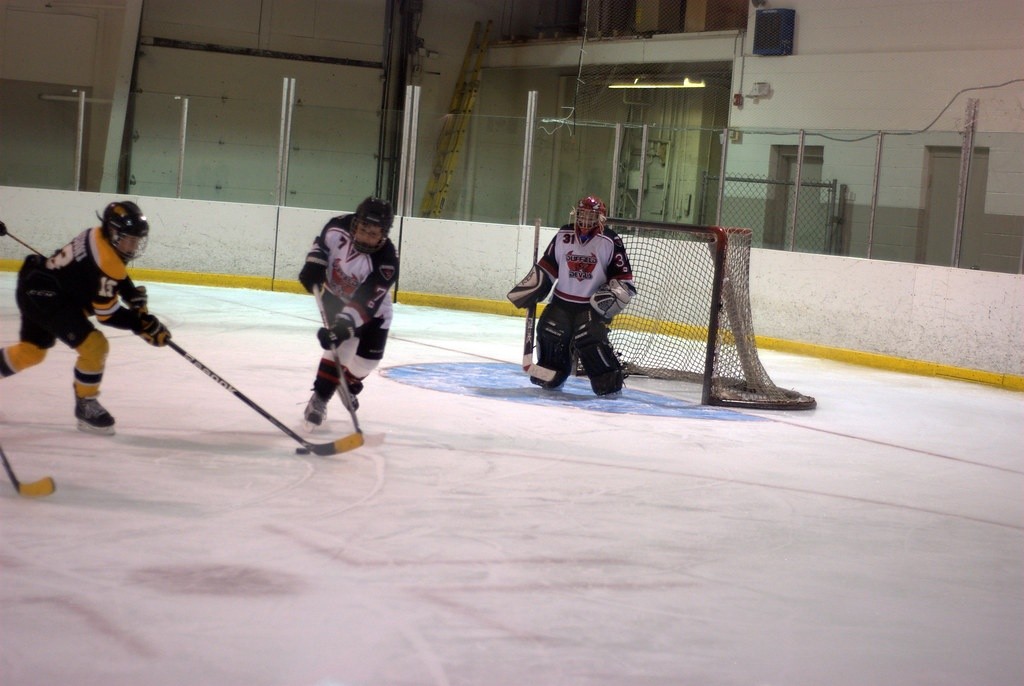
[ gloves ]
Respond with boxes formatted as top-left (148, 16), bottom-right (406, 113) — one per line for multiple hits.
top-left (136, 315), bottom-right (171, 347)
top-left (300, 252), bottom-right (329, 293)
top-left (317, 319), bottom-right (355, 350)
top-left (122, 288), bottom-right (149, 317)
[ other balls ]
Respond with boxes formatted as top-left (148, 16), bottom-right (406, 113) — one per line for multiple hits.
top-left (296, 448), bottom-right (310, 456)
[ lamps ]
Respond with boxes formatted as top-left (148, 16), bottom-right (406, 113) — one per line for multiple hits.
top-left (608, 76), bottom-right (705, 88)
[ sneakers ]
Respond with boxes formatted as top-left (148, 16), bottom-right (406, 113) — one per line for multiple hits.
top-left (73, 383), bottom-right (116, 436)
top-left (338, 386), bottom-right (359, 411)
top-left (297, 388), bottom-right (328, 434)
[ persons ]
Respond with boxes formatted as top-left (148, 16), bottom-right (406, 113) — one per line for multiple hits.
top-left (298, 195), bottom-right (399, 432)
top-left (0, 202), bottom-right (172, 437)
top-left (507, 194), bottom-right (638, 399)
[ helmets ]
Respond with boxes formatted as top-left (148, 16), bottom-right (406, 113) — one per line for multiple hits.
top-left (356, 196), bottom-right (394, 231)
top-left (576, 196), bottom-right (607, 217)
top-left (104, 201), bottom-right (149, 237)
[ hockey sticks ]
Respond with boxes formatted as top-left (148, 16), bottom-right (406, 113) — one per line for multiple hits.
top-left (522, 219), bottom-right (556, 382)
top-left (0, 447), bottom-right (56, 498)
top-left (7, 230), bottom-right (47, 260)
top-left (312, 283), bottom-right (385, 447)
top-left (164, 337), bottom-right (365, 456)
